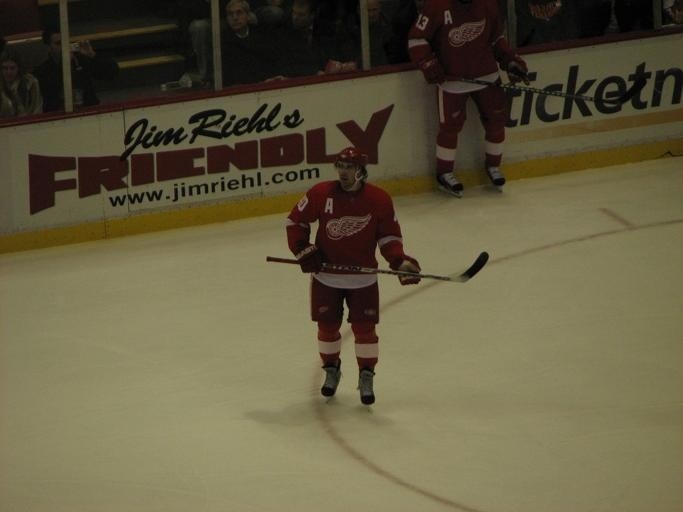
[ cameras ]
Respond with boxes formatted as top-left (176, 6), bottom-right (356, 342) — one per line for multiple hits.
top-left (70, 42), bottom-right (81, 52)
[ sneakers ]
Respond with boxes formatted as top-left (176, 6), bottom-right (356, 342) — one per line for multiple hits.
top-left (487, 165), bottom-right (504, 185)
top-left (320, 363), bottom-right (341, 398)
top-left (357, 369), bottom-right (379, 404)
top-left (436, 172), bottom-right (461, 191)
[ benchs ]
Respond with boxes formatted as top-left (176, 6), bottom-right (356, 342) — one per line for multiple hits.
top-left (0, 20), bottom-right (186, 71)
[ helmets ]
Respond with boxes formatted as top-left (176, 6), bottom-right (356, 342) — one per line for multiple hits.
top-left (333, 147), bottom-right (364, 164)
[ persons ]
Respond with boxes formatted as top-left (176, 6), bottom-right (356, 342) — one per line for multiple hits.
top-left (409, 0), bottom-right (527, 191)
top-left (32, 27), bottom-right (118, 113)
top-left (221, 0), bottom-right (408, 87)
top-left (1, 48), bottom-right (44, 119)
top-left (286, 147), bottom-right (421, 404)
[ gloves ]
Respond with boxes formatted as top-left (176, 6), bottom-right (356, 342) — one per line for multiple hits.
top-left (503, 54), bottom-right (528, 83)
top-left (294, 247), bottom-right (321, 271)
top-left (423, 63), bottom-right (443, 86)
top-left (395, 256), bottom-right (419, 285)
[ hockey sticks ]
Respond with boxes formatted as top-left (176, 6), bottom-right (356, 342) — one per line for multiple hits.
top-left (441, 76), bottom-right (647, 104)
top-left (266, 251), bottom-right (489, 283)
